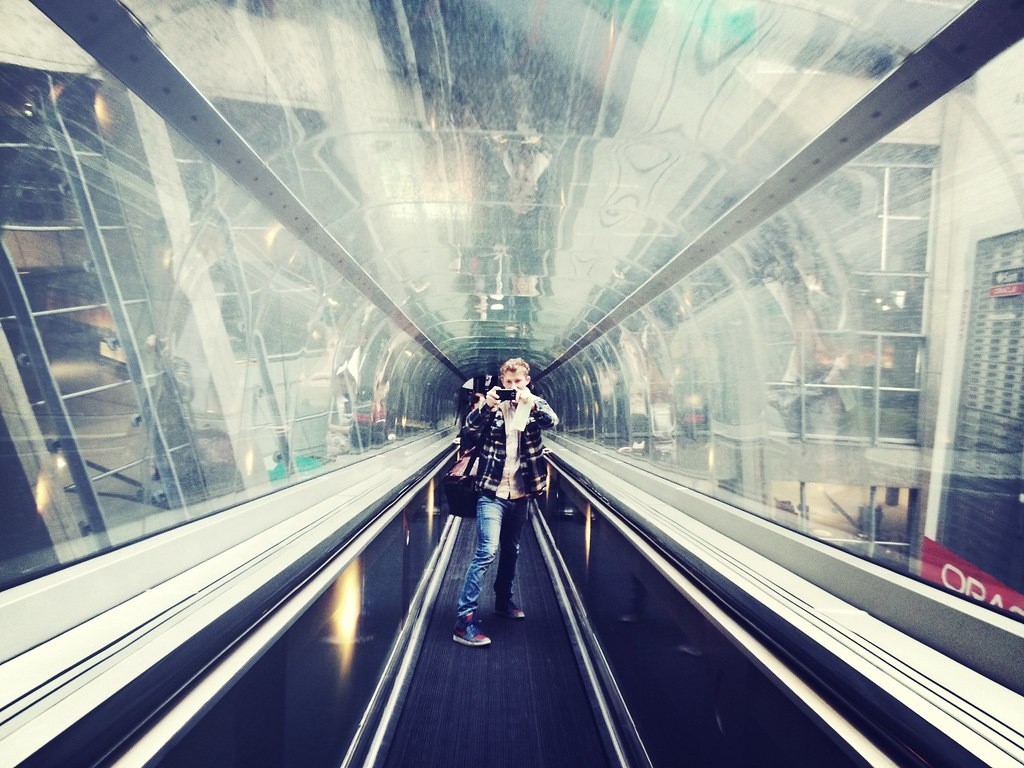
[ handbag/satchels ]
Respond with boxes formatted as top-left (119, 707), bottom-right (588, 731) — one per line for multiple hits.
top-left (446, 475), bottom-right (478, 518)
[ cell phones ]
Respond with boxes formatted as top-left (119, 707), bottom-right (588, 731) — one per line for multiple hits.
top-left (495, 389), bottom-right (516, 400)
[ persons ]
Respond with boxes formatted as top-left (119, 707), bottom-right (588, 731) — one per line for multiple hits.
top-left (452, 358), bottom-right (558, 647)
top-left (460, 393), bottom-right (485, 450)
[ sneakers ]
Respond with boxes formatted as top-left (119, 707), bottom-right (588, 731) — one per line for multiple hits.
top-left (453, 609), bottom-right (491, 646)
top-left (495, 606), bottom-right (525, 618)
